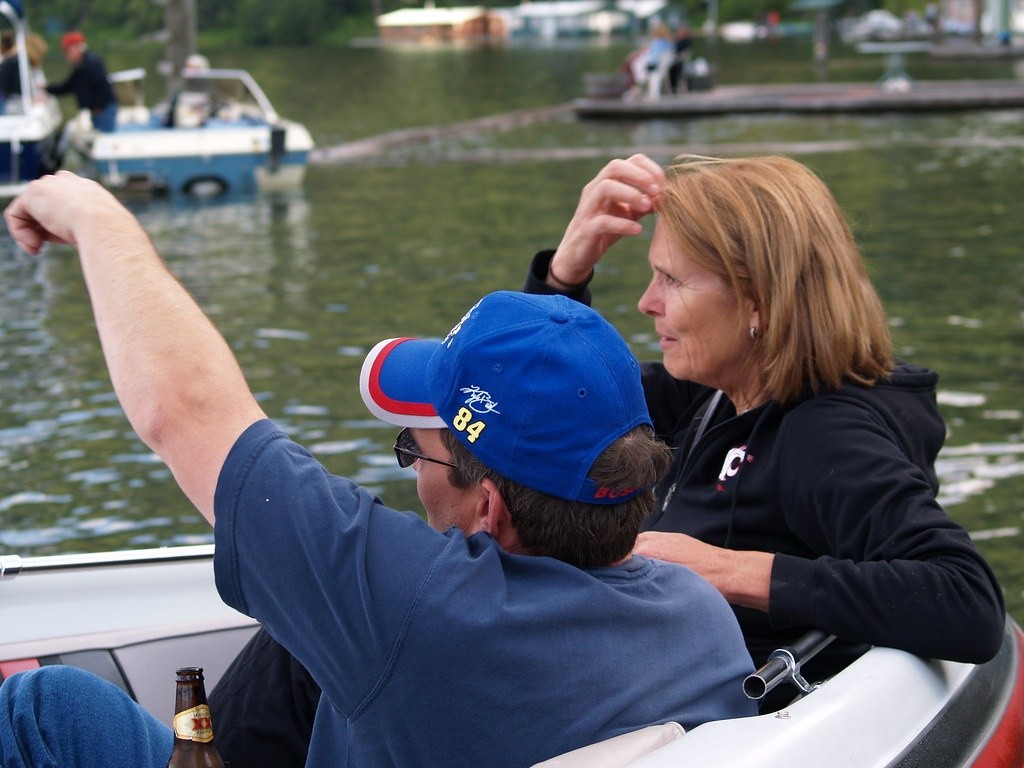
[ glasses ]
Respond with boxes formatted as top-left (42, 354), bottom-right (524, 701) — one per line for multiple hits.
top-left (393, 427), bottom-right (519, 525)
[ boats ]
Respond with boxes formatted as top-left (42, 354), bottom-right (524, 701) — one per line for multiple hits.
top-left (0, 544), bottom-right (1023, 768)
top-left (0, 0), bottom-right (316, 210)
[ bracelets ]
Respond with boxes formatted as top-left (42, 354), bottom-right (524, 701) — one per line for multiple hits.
top-left (549, 252), bottom-right (593, 288)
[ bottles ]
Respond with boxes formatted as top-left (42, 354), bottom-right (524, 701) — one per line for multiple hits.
top-left (167, 667), bottom-right (225, 768)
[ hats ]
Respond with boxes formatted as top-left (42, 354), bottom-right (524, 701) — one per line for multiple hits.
top-left (359, 291), bottom-right (656, 504)
top-left (59, 32), bottom-right (84, 48)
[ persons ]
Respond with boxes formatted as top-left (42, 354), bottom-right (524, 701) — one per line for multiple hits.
top-left (213, 149), bottom-right (1006, 767)
top-left (164, 53), bottom-right (222, 126)
top-left (625, 13), bottom-right (700, 105)
top-left (0, 25), bottom-right (120, 130)
top-left (0, 164), bottom-right (758, 768)
